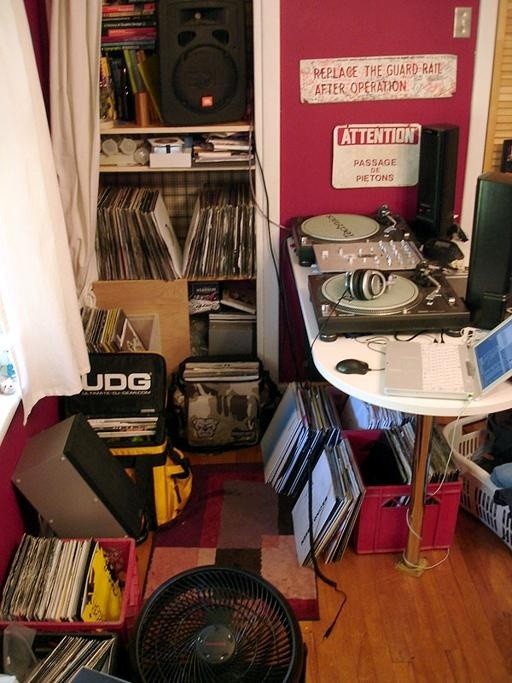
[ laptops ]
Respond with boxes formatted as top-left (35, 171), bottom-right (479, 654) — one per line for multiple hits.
top-left (384, 314), bottom-right (512, 401)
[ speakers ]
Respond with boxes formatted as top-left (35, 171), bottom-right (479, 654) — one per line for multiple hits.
top-left (467, 172), bottom-right (512, 327)
top-left (12, 413), bottom-right (148, 542)
top-left (416, 122), bottom-right (459, 239)
top-left (157, 0), bottom-right (252, 124)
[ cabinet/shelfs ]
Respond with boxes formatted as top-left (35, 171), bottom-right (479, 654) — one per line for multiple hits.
top-left (95, 120), bottom-right (257, 366)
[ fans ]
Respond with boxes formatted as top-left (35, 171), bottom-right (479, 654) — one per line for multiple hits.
top-left (133, 558), bottom-right (307, 682)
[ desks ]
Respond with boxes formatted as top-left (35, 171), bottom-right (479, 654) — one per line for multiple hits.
top-left (285, 219), bottom-right (512, 573)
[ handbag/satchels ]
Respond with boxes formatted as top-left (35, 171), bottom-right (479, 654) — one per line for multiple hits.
top-left (152, 445), bottom-right (193, 525)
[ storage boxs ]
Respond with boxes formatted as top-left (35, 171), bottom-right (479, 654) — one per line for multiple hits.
top-left (0, 537), bottom-right (140, 639)
top-left (57, 351), bottom-right (171, 529)
top-left (343, 428), bottom-right (464, 556)
top-left (0, 630), bottom-right (118, 682)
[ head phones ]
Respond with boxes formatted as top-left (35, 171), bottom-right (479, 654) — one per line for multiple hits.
top-left (345, 268), bottom-right (394, 301)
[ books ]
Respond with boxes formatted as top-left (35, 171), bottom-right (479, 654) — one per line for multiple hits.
top-left (1, 188), bottom-right (462, 682)
top-left (195, 132), bottom-right (254, 163)
top-left (97, 1), bottom-right (160, 126)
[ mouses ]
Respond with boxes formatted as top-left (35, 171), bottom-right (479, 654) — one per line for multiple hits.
top-left (337, 359), bottom-right (370, 374)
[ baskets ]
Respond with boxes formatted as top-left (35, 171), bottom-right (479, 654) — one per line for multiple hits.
top-left (443, 415), bottom-right (512, 551)
